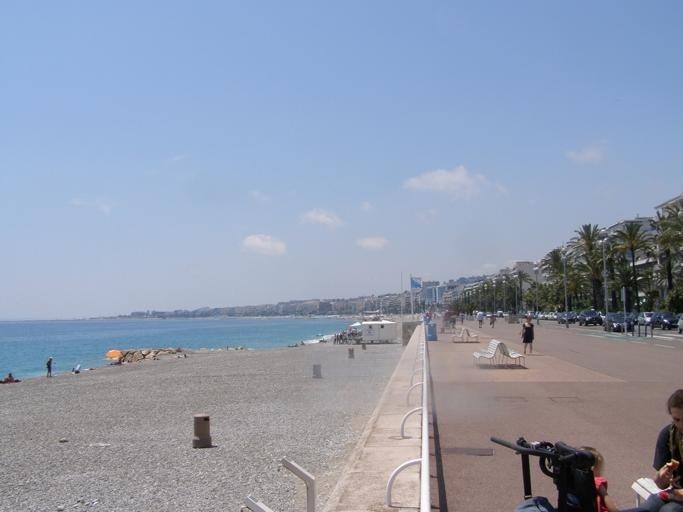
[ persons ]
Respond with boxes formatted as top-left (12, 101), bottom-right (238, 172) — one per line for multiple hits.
top-left (477, 312), bottom-right (483, 328)
top-left (521, 315), bottom-right (534, 356)
top-left (490, 312), bottom-right (496, 328)
top-left (580, 447), bottom-right (616, 510)
top-left (7, 373), bottom-right (21, 383)
top-left (333, 331), bottom-right (351, 344)
top-left (642, 387), bottom-right (683, 510)
top-left (46, 357), bottom-right (53, 377)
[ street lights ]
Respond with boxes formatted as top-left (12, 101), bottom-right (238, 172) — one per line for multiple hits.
top-left (532, 261), bottom-right (539, 326)
top-left (453, 273), bottom-right (518, 323)
top-left (596, 229), bottom-right (611, 332)
top-left (559, 247), bottom-right (569, 329)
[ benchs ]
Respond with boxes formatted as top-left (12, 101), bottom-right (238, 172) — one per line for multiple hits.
top-left (451, 328), bottom-right (480, 343)
top-left (472, 339), bottom-right (526, 370)
top-left (631, 477), bottom-right (670, 508)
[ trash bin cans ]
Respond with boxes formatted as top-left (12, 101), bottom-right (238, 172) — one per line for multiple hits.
top-left (361, 342), bottom-right (366, 349)
top-left (428, 323), bottom-right (437, 341)
top-left (313, 364), bottom-right (321, 378)
top-left (348, 347), bottom-right (355, 359)
top-left (193, 413), bottom-right (212, 449)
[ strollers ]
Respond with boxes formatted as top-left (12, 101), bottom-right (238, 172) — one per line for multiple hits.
top-left (488, 436), bottom-right (615, 512)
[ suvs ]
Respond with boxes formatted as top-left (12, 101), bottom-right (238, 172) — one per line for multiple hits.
top-left (556, 309), bottom-right (682, 333)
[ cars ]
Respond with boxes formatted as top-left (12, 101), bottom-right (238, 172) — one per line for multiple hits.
top-left (471, 309), bottom-right (557, 320)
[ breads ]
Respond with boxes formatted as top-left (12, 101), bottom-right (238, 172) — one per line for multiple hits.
top-left (667, 459), bottom-right (679, 470)
